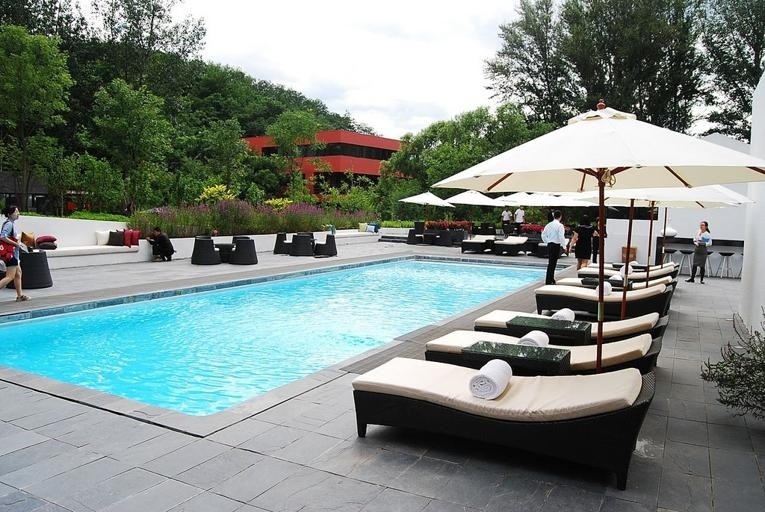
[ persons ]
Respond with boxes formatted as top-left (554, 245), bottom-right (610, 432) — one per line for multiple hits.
top-left (501, 205), bottom-right (512, 237)
top-left (686, 221), bottom-right (712, 284)
top-left (0, 204), bottom-right (30, 303)
top-left (542, 210), bottom-right (568, 285)
top-left (146, 227), bottom-right (175, 262)
top-left (513, 205), bottom-right (527, 235)
top-left (568, 215), bottom-right (600, 271)
top-left (593, 216), bottom-right (606, 263)
top-left (547, 207), bottom-right (555, 223)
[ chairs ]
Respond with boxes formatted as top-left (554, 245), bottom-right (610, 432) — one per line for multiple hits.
top-left (554, 260), bottom-right (679, 294)
top-left (5, 250), bottom-right (53, 289)
top-left (424, 329), bottom-right (663, 383)
top-left (352, 356), bottom-right (656, 492)
top-left (407, 218), bottom-right (582, 254)
top-left (473, 308), bottom-right (670, 343)
top-left (534, 281), bottom-right (673, 317)
top-left (190, 231), bottom-right (338, 266)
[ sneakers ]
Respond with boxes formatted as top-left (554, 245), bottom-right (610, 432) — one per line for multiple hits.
top-left (151, 257), bottom-right (163, 262)
top-left (16, 294), bottom-right (32, 302)
top-left (685, 277), bottom-right (705, 284)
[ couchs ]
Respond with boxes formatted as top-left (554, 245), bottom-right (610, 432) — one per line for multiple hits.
top-left (1, 216), bottom-right (139, 276)
top-left (333, 230), bottom-right (379, 246)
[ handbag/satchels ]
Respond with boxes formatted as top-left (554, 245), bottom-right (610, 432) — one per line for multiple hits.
top-left (0, 236), bottom-right (18, 261)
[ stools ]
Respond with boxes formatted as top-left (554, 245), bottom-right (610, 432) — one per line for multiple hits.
top-left (703, 249), bottom-right (713, 278)
top-left (664, 248), bottom-right (677, 263)
top-left (715, 251), bottom-right (734, 279)
top-left (678, 249), bottom-right (695, 277)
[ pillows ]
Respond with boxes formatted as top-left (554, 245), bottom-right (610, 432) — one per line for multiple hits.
top-left (38, 240), bottom-right (58, 250)
top-left (95, 228), bottom-right (109, 245)
top-left (34, 234), bottom-right (56, 243)
top-left (355, 220), bottom-right (380, 233)
top-left (122, 229), bottom-right (132, 248)
top-left (107, 230), bottom-right (125, 245)
top-left (18, 229), bottom-right (34, 250)
top-left (129, 230), bottom-right (139, 246)
